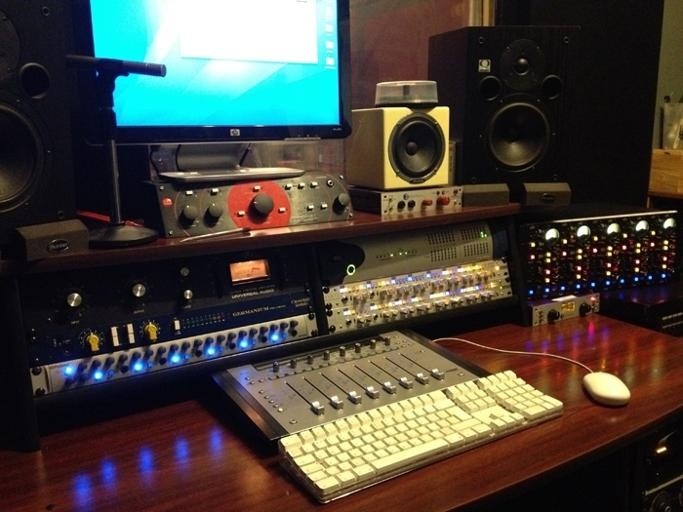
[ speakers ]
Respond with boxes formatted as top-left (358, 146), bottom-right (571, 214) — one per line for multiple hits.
top-left (0, 0), bottom-right (77, 261)
top-left (343, 104), bottom-right (451, 192)
top-left (428, 25), bottom-right (577, 201)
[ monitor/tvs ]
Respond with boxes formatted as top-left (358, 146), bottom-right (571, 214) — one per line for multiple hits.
top-left (86, 1), bottom-right (353, 143)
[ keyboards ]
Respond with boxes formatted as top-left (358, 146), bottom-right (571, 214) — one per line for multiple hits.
top-left (278, 369), bottom-right (564, 505)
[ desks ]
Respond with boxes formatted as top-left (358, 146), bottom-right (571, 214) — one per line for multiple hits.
top-left (1, 304), bottom-right (681, 509)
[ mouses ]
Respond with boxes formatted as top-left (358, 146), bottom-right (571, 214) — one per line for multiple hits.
top-left (581, 370), bottom-right (631, 408)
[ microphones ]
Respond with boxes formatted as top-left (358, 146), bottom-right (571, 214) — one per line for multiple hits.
top-left (65, 55), bottom-right (167, 77)
